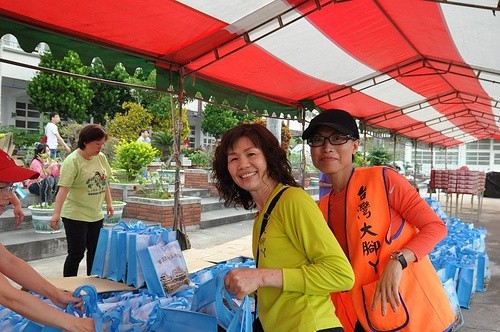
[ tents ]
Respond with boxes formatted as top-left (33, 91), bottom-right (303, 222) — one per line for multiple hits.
top-left (0, 0), bottom-right (500, 228)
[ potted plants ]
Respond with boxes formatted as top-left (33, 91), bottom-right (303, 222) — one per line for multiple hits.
top-left (25, 141), bottom-right (63, 233)
top-left (103, 131), bottom-right (226, 230)
top-left (301, 165), bottom-right (310, 188)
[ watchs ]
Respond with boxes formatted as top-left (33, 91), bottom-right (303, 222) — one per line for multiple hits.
top-left (389, 250), bottom-right (408, 270)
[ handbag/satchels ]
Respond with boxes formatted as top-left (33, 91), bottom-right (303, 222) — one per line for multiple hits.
top-left (0, 219), bottom-right (260, 332)
top-left (419, 196), bottom-right (492, 331)
top-left (40, 133), bottom-right (47, 145)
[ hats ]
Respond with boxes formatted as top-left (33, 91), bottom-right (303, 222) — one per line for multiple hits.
top-left (0, 148), bottom-right (41, 183)
top-left (301, 108), bottom-right (359, 140)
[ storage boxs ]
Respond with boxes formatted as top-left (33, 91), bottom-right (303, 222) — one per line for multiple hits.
top-left (430, 169), bottom-right (486, 194)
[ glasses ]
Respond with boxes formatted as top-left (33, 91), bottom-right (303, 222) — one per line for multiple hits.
top-left (306, 132), bottom-right (357, 147)
top-left (1, 185), bottom-right (18, 193)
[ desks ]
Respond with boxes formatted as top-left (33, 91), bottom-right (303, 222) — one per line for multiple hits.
top-left (428, 187), bottom-right (484, 222)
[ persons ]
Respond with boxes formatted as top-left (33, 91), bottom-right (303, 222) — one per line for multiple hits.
top-left (212, 122), bottom-right (356, 332)
top-left (137, 129), bottom-right (152, 177)
top-left (302, 109), bottom-right (456, 332)
top-left (8, 191), bottom-right (24, 226)
top-left (45, 112), bottom-right (71, 157)
top-left (319, 153), bottom-right (355, 197)
top-left (0, 147), bottom-right (94, 332)
top-left (50, 125), bottom-right (114, 277)
top-left (184, 137), bottom-right (189, 147)
top-left (27, 144), bottom-right (60, 202)
